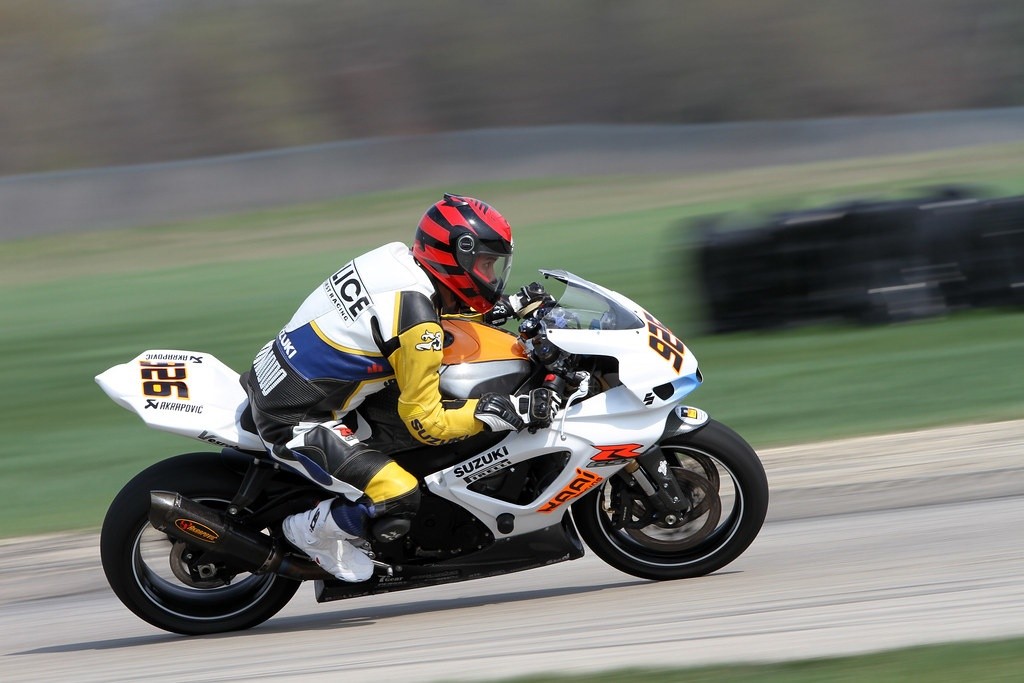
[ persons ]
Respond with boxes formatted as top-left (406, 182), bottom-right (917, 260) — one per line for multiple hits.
top-left (246, 192), bottom-right (561, 583)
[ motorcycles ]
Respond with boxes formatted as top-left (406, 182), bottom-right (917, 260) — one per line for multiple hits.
top-left (94, 268), bottom-right (769, 637)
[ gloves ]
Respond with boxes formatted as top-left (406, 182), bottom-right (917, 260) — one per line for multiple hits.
top-left (474, 388), bottom-right (560, 434)
top-left (488, 281), bottom-right (555, 328)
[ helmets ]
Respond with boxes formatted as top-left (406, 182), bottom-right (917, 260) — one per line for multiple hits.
top-left (413, 193), bottom-right (514, 315)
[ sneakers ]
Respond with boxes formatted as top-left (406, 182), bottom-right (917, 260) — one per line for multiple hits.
top-left (282, 505), bottom-right (374, 583)
